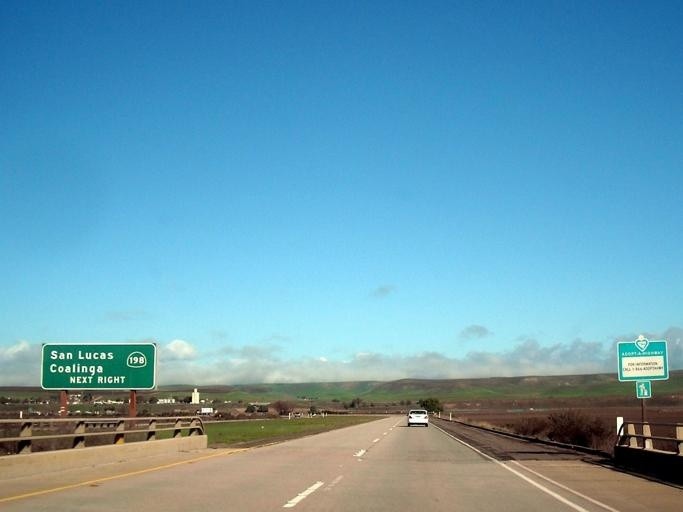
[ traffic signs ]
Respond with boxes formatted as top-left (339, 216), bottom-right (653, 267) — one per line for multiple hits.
top-left (39, 341), bottom-right (156, 391)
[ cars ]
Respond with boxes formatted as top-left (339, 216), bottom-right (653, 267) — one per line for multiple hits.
top-left (408, 408), bottom-right (428, 428)
